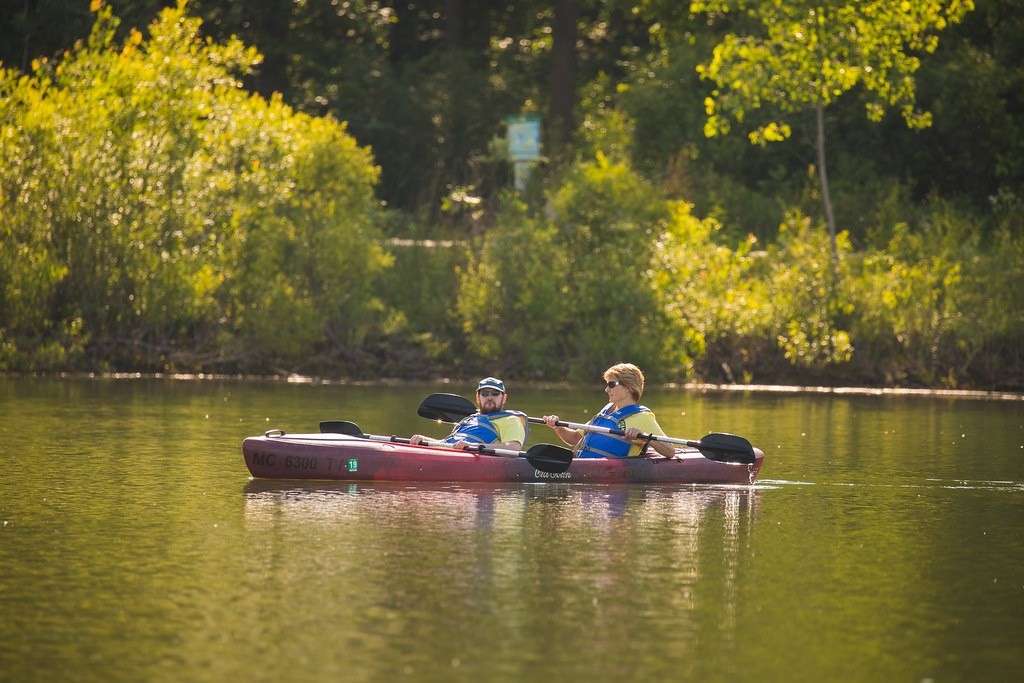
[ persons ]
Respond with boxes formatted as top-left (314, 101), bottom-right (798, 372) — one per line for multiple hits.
top-left (543, 363), bottom-right (675, 459)
top-left (410, 377), bottom-right (526, 452)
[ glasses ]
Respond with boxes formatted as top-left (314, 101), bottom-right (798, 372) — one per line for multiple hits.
top-left (604, 381), bottom-right (622, 388)
top-left (480, 389), bottom-right (500, 396)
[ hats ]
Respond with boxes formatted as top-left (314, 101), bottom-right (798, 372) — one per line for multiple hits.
top-left (476, 377), bottom-right (505, 393)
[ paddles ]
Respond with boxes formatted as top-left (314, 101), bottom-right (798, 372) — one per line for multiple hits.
top-left (319, 420), bottom-right (573, 474)
top-left (418, 393), bottom-right (756, 463)
